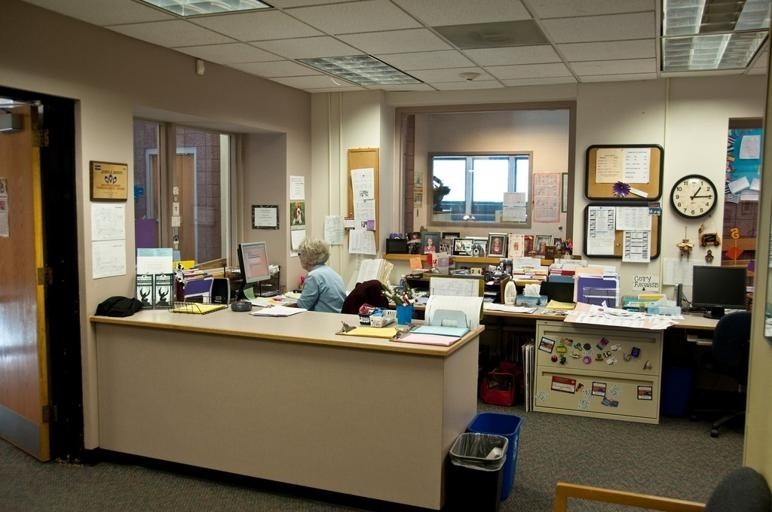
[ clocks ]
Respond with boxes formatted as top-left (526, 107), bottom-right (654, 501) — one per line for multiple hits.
top-left (668, 172), bottom-right (719, 222)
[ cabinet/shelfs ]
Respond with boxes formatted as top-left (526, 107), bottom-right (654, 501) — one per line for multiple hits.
top-left (533, 319), bottom-right (661, 428)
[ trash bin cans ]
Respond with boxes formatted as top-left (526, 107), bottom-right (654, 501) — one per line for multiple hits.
top-left (466, 411), bottom-right (524, 500)
top-left (449, 432), bottom-right (509, 505)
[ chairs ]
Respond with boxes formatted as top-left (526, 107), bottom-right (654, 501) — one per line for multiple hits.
top-left (699, 312), bottom-right (751, 437)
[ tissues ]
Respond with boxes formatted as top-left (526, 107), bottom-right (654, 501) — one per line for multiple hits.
top-left (513, 284), bottom-right (548, 309)
top-left (644, 296), bottom-right (681, 318)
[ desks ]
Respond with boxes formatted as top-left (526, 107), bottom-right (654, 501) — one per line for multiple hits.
top-left (342, 287), bottom-right (752, 425)
top-left (88, 299), bottom-right (486, 511)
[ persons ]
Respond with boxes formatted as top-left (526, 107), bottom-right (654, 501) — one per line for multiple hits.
top-left (538, 241), bottom-right (546, 255)
top-left (492, 238), bottom-right (502, 254)
top-left (295, 237), bottom-right (346, 314)
top-left (423, 238), bottom-right (436, 253)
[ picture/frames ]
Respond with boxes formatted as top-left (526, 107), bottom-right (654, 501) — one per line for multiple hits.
top-left (452, 237), bottom-right (474, 255)
top-left (521, 235), bottom-right (534, 255)
top-left (251, 204), bottom-right (279, 231)
top-left (484, 231), bottom-right (506, 259)
top-left (438, 238), bottom-right (452, 255)
top-left (507, 233), bottom-right (524, 259)
top-left (420, 230), bottom-right (440, 256)
top-left (535, 234), bottom-right (553, 257)
top-left (442, 231), bottom-right (461, 255)
top-left (89, 159), bottom-right (130, 204)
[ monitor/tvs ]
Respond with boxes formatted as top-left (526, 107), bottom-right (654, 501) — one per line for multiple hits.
top-left (236, 241), bottom-right (270, 301)
top-left (693, 266), bottom-right (746, 319)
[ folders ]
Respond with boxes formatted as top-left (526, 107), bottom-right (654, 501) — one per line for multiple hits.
top-left (408, 323), bottom-right (471, 337)
top-left (335, 320), bottom-right (406, 339)
top-left (389, 328), bottom-right (461, 347)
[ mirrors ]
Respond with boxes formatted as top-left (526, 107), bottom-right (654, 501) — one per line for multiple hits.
top-left (405, 98), bottom-right (577, 254)
